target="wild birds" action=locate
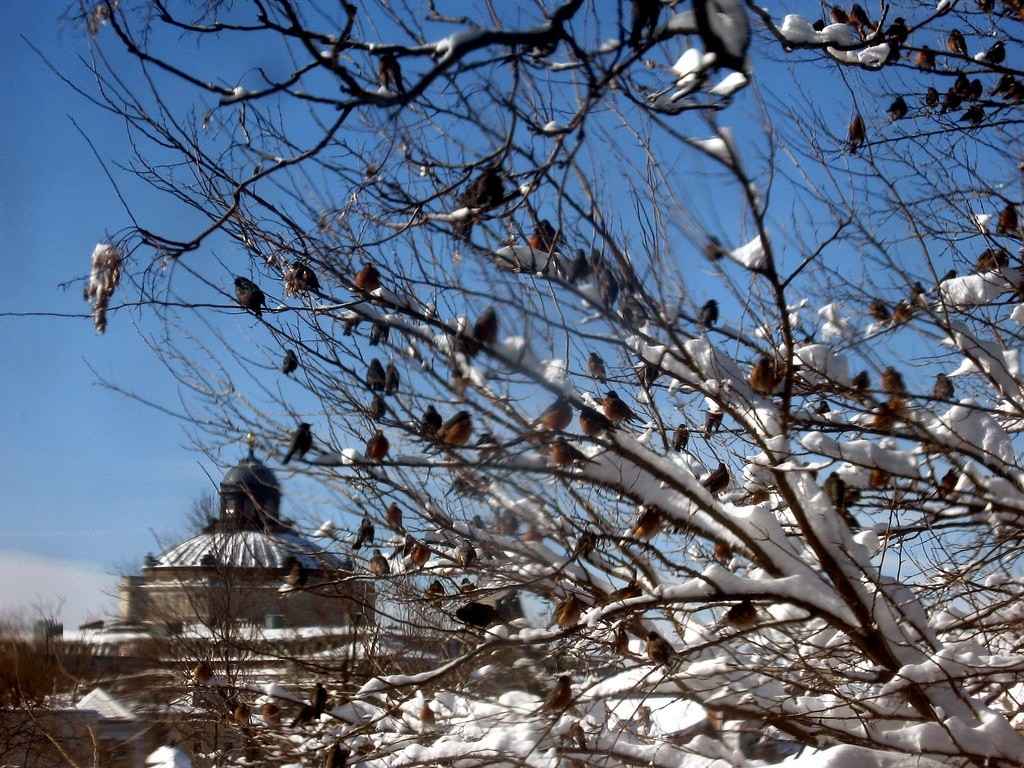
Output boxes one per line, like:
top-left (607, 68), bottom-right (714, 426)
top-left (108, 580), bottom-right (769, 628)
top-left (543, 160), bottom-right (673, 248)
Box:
top-left (231, 5), bottom-right (1023, 732)
top-left (193, 660), bottom-right (214, 686)
